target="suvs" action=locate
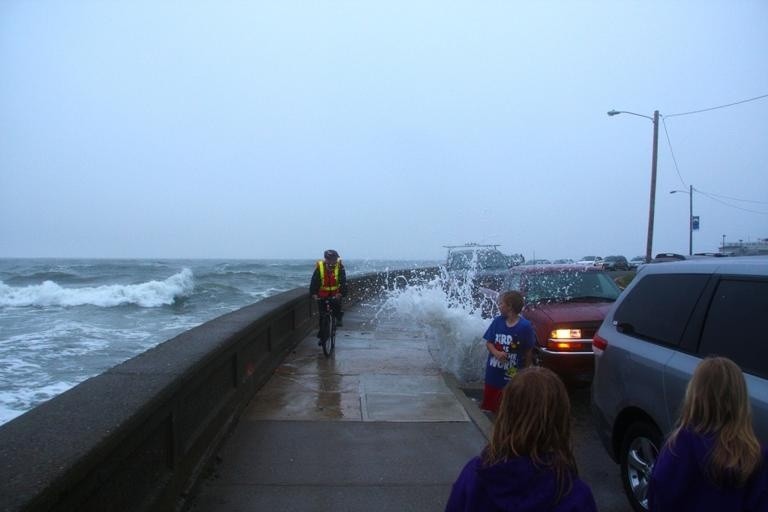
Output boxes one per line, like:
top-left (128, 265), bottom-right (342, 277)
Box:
top-left (592, 255), bottom-right (768, 512)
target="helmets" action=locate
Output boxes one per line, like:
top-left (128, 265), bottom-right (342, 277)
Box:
top-left (324, 250), bottom-right (339, 259)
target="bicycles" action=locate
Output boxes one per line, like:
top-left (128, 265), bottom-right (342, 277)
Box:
top-left (315, 297), bottom-right (336, 356)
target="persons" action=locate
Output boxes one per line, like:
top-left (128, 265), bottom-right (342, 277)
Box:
top-left (479, 290), bottom-right (536, 414)
top-left (310, 250), bottom-right (346, 344)
top-left (445, 366), bottom-right (597, 511)
top-left (648, 357), bottom-right (768, 511)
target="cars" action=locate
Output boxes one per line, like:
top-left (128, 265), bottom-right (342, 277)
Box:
top-left (446, 249), bottom-right (646, 375)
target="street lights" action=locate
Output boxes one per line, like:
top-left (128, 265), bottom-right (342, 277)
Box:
top-left (670, 190), bottom-right (692, 256)
top-left (608, 111), bottom-right (658, 263)
